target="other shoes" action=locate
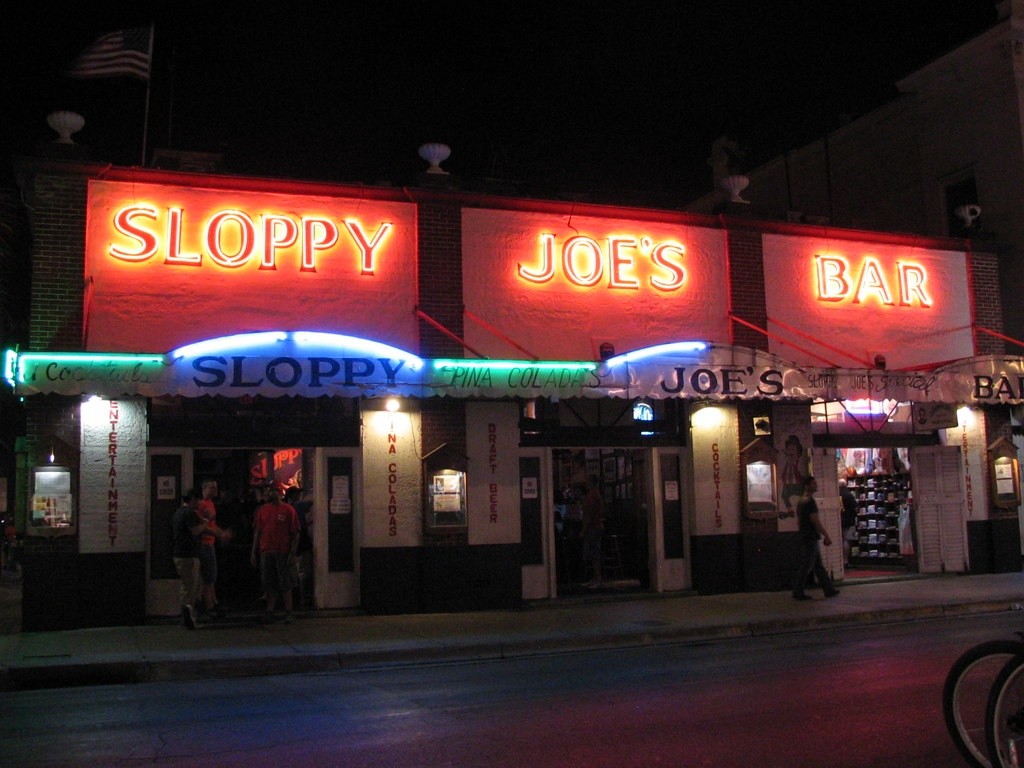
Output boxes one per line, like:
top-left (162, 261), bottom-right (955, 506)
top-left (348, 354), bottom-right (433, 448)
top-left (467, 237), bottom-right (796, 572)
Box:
top-left (265, 612), bottom-right (276, 623)
top-left (183, 603), bottom-right (199, 631)
top-left (284, 612), bottom-right (297, 623)
top-left (825, 589), bottom-right (841, 599)
top-left (208, 604), bottom-right (228, 622)
top-left (791, 592), bottom-right (812, 601)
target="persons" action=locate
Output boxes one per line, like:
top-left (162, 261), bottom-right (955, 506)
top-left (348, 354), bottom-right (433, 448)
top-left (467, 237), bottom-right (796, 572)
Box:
top-left (579, 475), bottom-right (604, 587)
top-left (791, 474), bottom-right (840, 600)
top-left (839, 479), bottom-right (856, 567)
top-left (172, 478), bottom-right (314, 629)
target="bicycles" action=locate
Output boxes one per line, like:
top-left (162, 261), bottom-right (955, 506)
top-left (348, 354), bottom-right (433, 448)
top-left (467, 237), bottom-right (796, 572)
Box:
top-left (941, 632), bottom-right (1024, 768)
top-left (982, 652), bottom-right (1024, 768)
